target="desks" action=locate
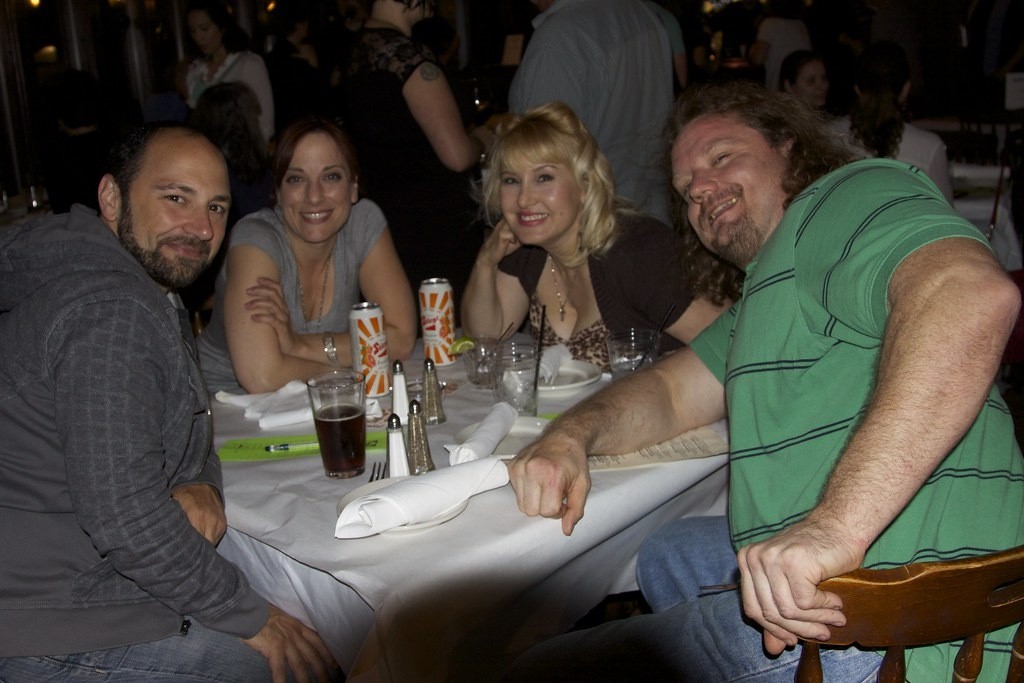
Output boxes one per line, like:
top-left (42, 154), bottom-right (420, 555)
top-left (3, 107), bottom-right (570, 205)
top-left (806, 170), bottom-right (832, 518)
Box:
top-left (910, 117), bottom-right (1021, 168)
top-left (939, 160), bottom-right (1023, 274)
top-left (185, 327), bottom-right (734, 683)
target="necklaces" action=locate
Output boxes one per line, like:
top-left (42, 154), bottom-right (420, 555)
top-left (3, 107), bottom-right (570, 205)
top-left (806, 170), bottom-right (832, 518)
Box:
top-left (551, 267), bottom-right (575, 322)
top-left (295, 255), bottom-right (332, 334)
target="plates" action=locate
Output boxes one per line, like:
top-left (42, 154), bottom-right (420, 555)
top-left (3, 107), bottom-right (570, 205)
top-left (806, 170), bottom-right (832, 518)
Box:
top-left (336, 474), bottom-right (470, 535)
top-left (536, 358), bottom-right (604, 398)
top-left (452, 416), bottom-right (552, 458)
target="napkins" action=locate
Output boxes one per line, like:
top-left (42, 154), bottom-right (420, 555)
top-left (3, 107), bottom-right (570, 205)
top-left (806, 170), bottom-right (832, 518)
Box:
top-left (332, 455), bottom-right (508, 541)
top-left (504, 344), bottom-right (573, 394)
top-left (242, 379), bottom-right (384, 429)
top-left (442, 400), bottom-right (517, 466)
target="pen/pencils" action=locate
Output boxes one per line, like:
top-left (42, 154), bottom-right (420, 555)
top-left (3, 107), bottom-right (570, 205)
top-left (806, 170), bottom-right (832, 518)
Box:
top-left (265, 442), bottom-right (319, 452)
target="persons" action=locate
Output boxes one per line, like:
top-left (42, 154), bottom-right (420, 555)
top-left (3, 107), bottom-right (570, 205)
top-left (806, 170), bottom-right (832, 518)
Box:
top-left (0, 122), bottom-right (347, 682)
top-left (824, 41), bottom-right (954, 206)
top-left (779, 51), bottom-right (828, 106)
top-left (199, 120), bottom-right (417, 395)
top-left (319, 0), bottom-right (500, 304)
top-left (750, 0), bottom-right (810, 91)
top-left (509, 1), bottom-right (706, 230)
top-left (184, 0), bottom-right (273, 149)
top-left (705, 0), bottom-right (765, 78)
top-left (266, 0), bottom-right (325, 136)
top-left (495, 76), bottom-right (1024, 683)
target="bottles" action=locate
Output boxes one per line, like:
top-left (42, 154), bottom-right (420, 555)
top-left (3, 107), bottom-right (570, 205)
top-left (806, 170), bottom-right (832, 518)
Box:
top-left (488, 345), bottom-right (539, 413)
top-left (386, 414), bottom-right (410, 479)
top-left (423, 357), bottom-right (446, 425)
top-left (392, 358), bottom-right (409, 425)
top-left (406, 400), bottom-right (436, 474)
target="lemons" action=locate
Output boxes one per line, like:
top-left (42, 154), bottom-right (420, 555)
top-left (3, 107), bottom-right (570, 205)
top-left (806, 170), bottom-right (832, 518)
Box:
top-left (448, 337), bottom-right (476, 355)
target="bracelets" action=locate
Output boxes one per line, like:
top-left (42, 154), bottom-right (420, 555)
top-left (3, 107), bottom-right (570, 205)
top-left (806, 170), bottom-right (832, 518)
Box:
top-left (323, 330), bottom-right (337, 362)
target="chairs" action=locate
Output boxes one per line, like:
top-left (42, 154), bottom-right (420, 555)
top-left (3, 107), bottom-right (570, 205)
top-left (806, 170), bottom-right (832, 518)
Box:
top-left (793, 545), bottom-right (1024, 683)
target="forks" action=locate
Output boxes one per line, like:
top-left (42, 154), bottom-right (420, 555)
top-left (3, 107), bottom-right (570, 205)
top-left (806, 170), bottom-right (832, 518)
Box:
top-left (368, 462), bottom-right (386, 482)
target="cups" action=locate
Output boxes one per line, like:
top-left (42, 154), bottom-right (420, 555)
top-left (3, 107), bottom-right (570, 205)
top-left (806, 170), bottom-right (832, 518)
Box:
top-left (605, 325), bottom-right (665, 382)
top-left (305, 370), bottom-right (367, 480)
top-left (465, 334), bottom-right (502, 387)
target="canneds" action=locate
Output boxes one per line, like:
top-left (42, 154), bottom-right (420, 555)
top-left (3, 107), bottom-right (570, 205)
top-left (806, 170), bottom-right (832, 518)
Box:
top-left (418, 278), bottom-right (457, 367)
top-left (348, 302), bottom-right (392, 397)
